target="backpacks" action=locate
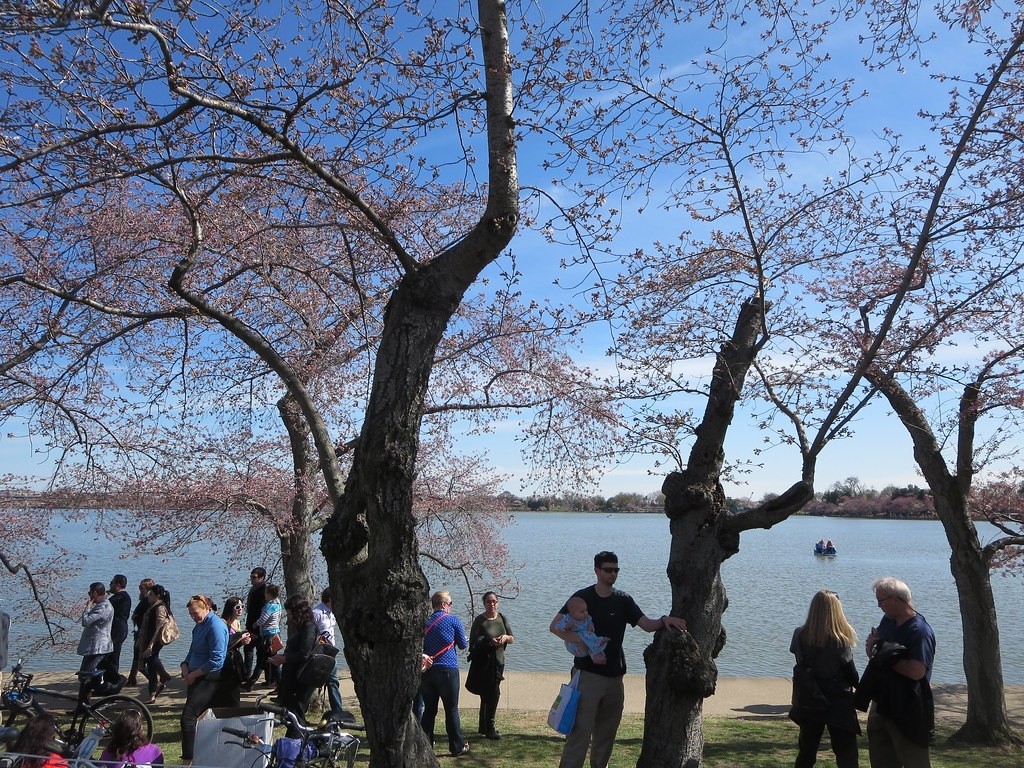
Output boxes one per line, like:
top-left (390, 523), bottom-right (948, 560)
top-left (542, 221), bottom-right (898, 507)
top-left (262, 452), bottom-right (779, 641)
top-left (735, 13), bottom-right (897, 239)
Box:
top-left (294, 621), bottom-right (339, 687)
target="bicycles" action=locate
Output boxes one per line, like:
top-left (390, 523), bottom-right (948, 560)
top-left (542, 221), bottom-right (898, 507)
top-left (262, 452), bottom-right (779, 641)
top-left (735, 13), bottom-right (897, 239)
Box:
top-left (814, 543), bottom-right (836, 555)
top-left (0, 658), bottom-right (153, 757)
top-left (221, 703), bottom-right (359, 768)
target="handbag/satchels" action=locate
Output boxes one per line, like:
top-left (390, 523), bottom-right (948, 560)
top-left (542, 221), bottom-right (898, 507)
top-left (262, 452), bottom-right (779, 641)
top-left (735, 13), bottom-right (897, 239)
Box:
top-left (547, 670), bottom-right (581, 736)
top-left (421, 654), bottom-right (433, 672)
top-left (155, 603), bottom-right (179, 646)
top-left (266, 634), bottom-right (284, 658)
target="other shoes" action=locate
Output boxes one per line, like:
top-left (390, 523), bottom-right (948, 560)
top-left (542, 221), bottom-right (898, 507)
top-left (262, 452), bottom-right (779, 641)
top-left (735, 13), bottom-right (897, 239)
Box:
top-left (144, 696), bottom-right (155, 704)
top-left (481, 730), bottom-right (500, 740)
top-left (125, 675), bottom-right (136, 686)
top-left (453, 742), bottom-right (470, 756)
top-left (155, 684), bottom-right (166, 699)
top-left (66, 708), bottom-right (92, 718)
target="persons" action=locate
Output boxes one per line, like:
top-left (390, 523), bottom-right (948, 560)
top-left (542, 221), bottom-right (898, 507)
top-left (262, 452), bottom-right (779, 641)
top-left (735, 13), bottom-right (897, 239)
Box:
top-left (11, 713), bottom-right (70, 768)
top-left (283, 586), bottom-right (343, 738)
top-left (413, 590), bottom-right (470, 757)
top-left (179, 593), bottom-right (229, 768)
top-left (0, 609), bottom-right (12, 670)
top-left (221, 567), bottom-right (283, 692)
top-left (548, 551), bottom-right (688, 768)
top-left (789, 590), bottom-right (860, 768)
top-left (813, 539), bottom-right (837, 556)
top-left (66, 574), bottom-right (170, 717)
top-left (99, 710), bottom-right (165, 768)
top-left (865, 578), bottom-right (938, 768)
top-left (468, 591), bottom-right (514, 741)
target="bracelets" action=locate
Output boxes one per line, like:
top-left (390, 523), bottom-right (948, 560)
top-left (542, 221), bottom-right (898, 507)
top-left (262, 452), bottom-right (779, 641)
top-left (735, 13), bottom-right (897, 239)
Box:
top-left (658, 613), bottom-right (668, 625)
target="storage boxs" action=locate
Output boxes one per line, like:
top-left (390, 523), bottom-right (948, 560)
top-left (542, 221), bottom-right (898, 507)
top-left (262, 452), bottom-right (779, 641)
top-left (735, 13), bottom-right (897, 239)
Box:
top-left (194, 707), bottom-right (275, 768)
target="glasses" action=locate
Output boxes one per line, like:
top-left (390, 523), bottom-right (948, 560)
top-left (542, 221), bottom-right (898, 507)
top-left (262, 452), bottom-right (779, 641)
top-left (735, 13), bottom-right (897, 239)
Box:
top-left (485, 601), bottom-right (498, 604)
top-left (250, 575), bottom-right (257, 580)
top-left (88, 592), bottom-right (93, 596)
top-left (191, 595), bottom-right (206, 606)
top-left (830, 591), bottom-right (839, 600)
top-left (232, 604), bottom-right (245, 610)
top-left (129, 709), bottom-right (141, 716)
top-left (444, 601), bottom-right (453, 605)
top-left (601, 567), bottom-right (620, 573)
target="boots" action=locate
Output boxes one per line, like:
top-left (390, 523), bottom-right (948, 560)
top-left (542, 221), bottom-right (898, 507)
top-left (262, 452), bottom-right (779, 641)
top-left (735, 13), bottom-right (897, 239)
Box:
top-left (158, 669), bottom-right (172, 684)
